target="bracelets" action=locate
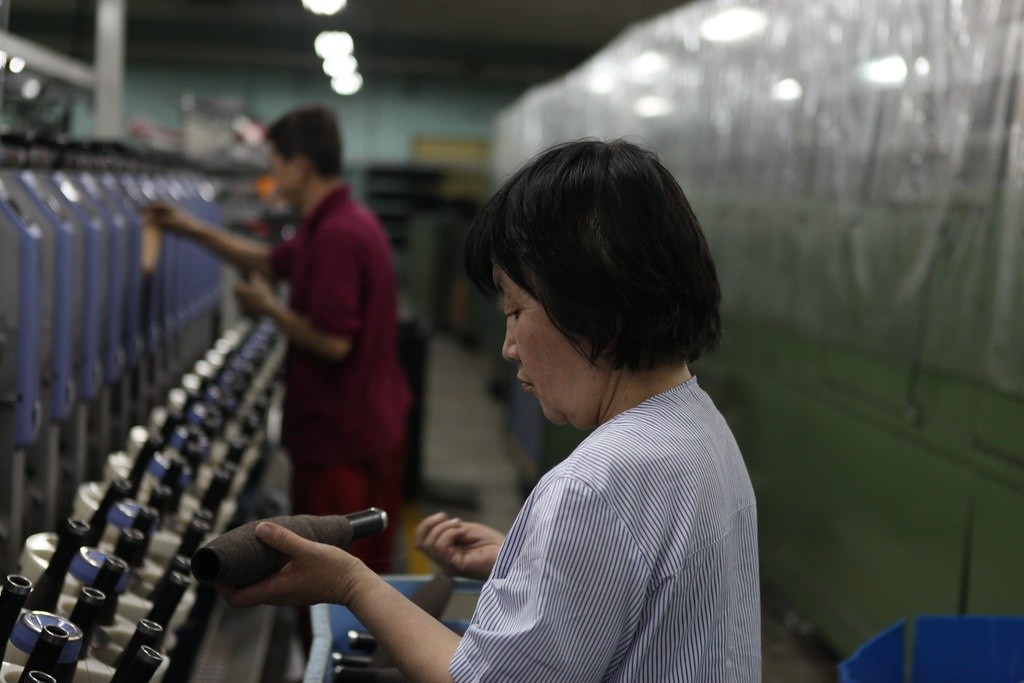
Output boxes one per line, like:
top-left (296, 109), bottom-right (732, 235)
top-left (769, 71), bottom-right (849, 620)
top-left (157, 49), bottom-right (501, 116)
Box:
top-left (269, 296), bottom-right (289, 327)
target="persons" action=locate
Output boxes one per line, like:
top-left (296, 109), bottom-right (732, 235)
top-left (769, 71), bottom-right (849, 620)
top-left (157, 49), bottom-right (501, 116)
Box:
top-left (146, 107), bottom-right (411, 683)
top-left (216, 138), bottom-right (762, 683)
